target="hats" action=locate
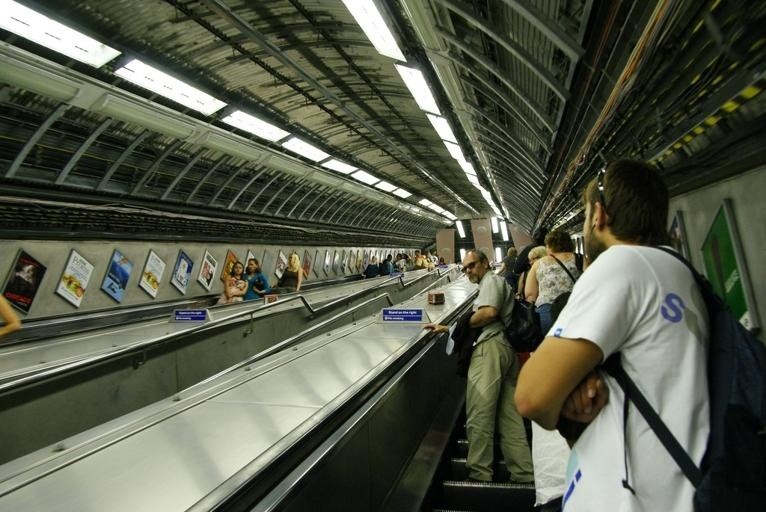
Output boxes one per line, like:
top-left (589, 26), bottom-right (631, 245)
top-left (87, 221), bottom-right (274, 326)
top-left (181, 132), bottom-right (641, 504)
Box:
top-left (533, 227), bottom-right (550, 243)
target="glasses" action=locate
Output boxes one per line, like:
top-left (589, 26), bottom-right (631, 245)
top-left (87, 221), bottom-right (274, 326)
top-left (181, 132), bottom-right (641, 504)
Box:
top-left (597, 159), bottom-right (611, 215)
top-left (461, 259), bottom-right (481, 273)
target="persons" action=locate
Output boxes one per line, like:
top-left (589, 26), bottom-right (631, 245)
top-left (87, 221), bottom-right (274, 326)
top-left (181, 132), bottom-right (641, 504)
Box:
top-left (273, 253), bottom-right (303, 293)
top-left (217, 261), bottom-right (248, 305)
top-left (0, 295), bottom-right (21, 337)
top-left (514, 161), bottom-right (710, 512)
top-left (424, 249), bottom-right (534, 483)
top-left (497, 227), bottom-right (589, 507)
top-left (243, 259), bottom-right (271, 300)
top-left (363, 249), bottom-right (448, 279)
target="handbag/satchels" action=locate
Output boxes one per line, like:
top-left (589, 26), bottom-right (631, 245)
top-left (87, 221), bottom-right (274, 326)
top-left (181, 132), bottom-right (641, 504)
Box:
top-left (694, 275), bottom-right (766, 511)
top-left (504, 297), bottom-right (543, 352)
top-left (254, 278), bottom-right (265, 297)
